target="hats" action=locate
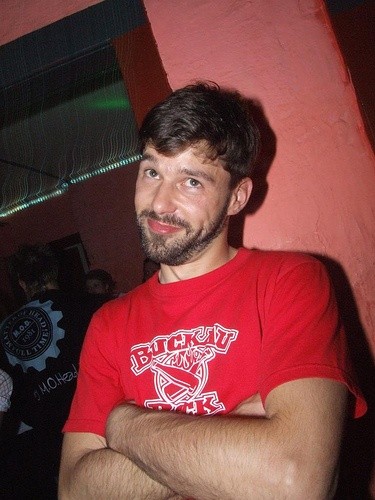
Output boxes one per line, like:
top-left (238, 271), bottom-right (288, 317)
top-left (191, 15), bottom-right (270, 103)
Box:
top-left (0, 368), bottom-right (13, 412)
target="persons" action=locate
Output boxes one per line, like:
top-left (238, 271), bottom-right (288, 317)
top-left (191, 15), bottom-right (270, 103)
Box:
top-left (1, 242), bottom-right (111, 445)
top-left (142, 257), bottom-right (161, 284)
top-left (59, 78), bottom-right (370, 500)
top-left (82, 267), bottom-right (129, 298)
